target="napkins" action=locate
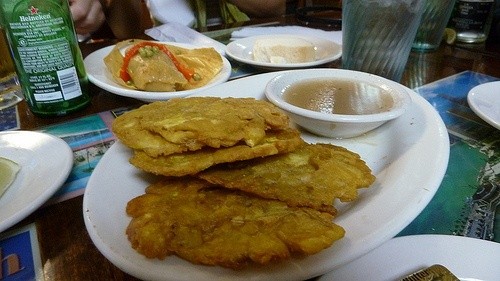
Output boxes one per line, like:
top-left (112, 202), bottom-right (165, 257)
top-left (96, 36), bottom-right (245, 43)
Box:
top-left (144, 20), bottom-right (227, 59)
top-left (230, 26), bottom-right (342, 46)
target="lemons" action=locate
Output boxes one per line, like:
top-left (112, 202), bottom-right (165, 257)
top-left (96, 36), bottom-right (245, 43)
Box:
top-left (0, 157), bottom-right (22, 197)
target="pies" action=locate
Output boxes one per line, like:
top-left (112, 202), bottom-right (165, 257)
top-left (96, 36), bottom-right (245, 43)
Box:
top-left (112, 96), bottom-right (374, 267)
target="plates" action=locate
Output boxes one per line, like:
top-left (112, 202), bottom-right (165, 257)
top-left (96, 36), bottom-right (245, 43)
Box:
top-left (467, 80), bottom-right (500, 130)
top-left (83, 41), bottom-right (232, 104)
top-left (81, 68), bottom-right (450, 281)
top-left (0, 130), bottom-right (74, 234)
top-left (224, 33), bottom-right (342, 71)
top-left (316, 233), bottom-right (500, 281)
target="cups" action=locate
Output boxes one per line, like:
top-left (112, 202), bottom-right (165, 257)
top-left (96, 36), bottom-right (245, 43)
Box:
top-left (340, 0), bottom-right (426, 84)
top-left (410, 0), bottom-right (456, 52)
top-left (450, 0), bottom-right (496, 44)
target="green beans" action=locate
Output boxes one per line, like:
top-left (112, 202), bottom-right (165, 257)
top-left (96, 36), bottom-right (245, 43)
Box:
top-left (127, 45), bottom-right (201, 90)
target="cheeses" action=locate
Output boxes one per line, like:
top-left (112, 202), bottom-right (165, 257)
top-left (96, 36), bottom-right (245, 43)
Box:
top-left (252, 35), bottom-right (314, 64)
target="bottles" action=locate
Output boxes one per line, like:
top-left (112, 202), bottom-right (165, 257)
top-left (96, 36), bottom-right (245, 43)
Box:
top-left (0, 0), bottom-right (94, 118)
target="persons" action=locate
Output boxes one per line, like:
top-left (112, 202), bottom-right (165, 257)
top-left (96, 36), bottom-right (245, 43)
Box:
top-left (0, 0), bottom-right (288, 73)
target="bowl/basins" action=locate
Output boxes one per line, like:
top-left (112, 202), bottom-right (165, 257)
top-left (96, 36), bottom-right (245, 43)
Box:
top-left (264, 68), bottom-right (413, 140)
